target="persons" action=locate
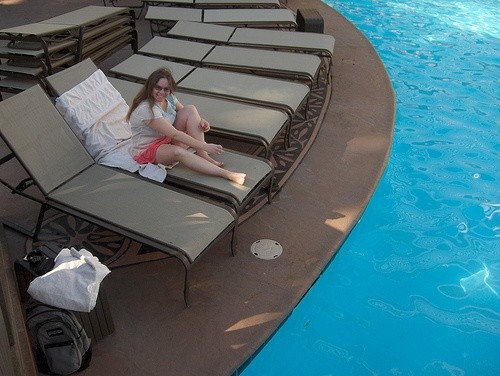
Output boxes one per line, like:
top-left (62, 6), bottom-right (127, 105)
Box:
top-left (123, 67), bottom-right (247, 185)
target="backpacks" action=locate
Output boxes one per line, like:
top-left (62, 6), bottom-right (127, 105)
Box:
top-left (23, 298), bottom-right (92, 376)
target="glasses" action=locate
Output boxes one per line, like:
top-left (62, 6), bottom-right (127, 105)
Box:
top-left (153, 85), bottom-right (171, 92)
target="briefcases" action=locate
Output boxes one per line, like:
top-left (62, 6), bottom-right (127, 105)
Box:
top-left (14, 242), bottom-right (114, 344)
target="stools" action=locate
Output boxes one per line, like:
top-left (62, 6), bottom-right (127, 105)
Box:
top-left (15, 251), bottom-right (115, 347)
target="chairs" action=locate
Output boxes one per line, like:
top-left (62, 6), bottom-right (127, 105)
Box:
top-left (0, 0), bottom-right (336, 167)
top-left (0, 83), bottom-right (236, 308)
top-left (46, 58), bottom-right (274, 255)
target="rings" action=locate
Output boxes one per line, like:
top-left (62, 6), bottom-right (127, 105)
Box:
top-left (214, 153), bottom-right (215, 155)
